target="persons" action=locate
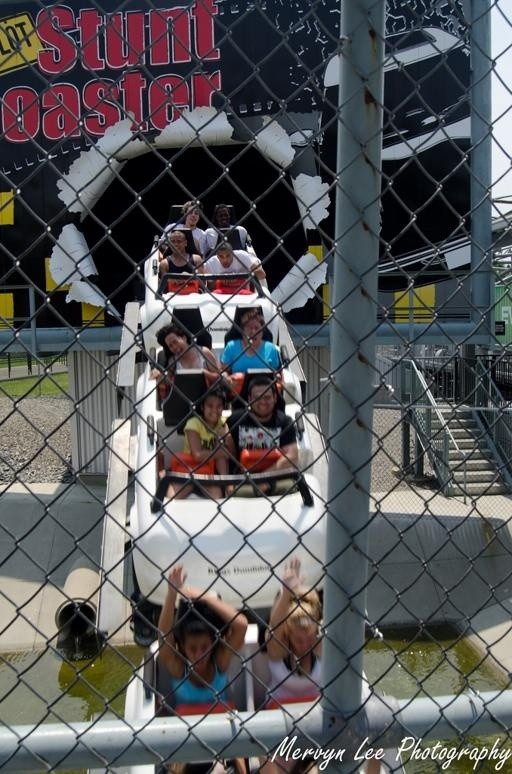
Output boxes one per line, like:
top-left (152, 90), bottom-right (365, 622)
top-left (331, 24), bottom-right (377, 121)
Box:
top-left (222, 310), bottom-right (281, 376)
top-left (200, 202), bottom-right (251, 248)
top-left (151, 322), bottom-right (216, 400)
top-left (166, 199), bottom-right (208, 251)
top-left (159, 226), bottom-right (205, 277)
top-left (259, 554), bottom-right (381, 773)
top-left (154, 562), bottom-right (251, 773)
top-left (171, 387), bottom-right (236, 498)
top-left (227, 377), bottom-right (302, 498)
top-left (204, 240), bottom-right (266, 277)
